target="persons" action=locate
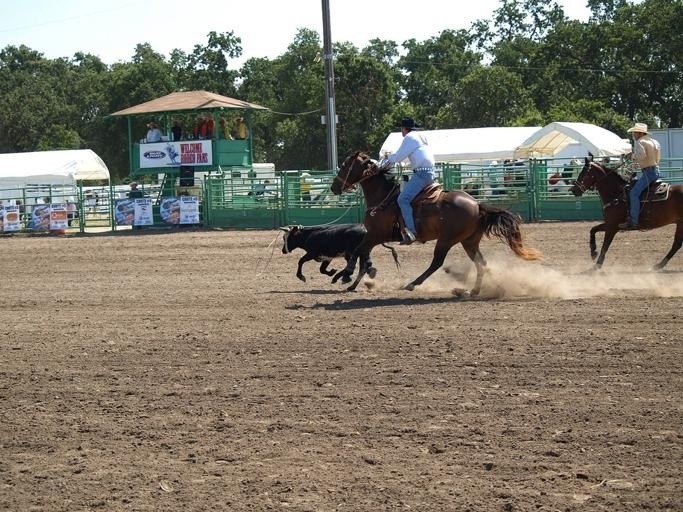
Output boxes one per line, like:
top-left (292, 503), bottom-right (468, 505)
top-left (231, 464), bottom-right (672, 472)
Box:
top-left (143, 115), bottom-right (249, 144)
top-left (248, 179), bottom-right (279, 209)
top-left (123, 211), bottom-right (135, 224)
top-left (115, 204), bottom-right (127, 225)
top-left (0, 187), bottom-right (99, 232)
top-left (299, 172), bottom-right (319, 208)
top-left (169, 145), bottom-right (176, 158)
top-left (163, 143), bottom-right (176, 166)
top-left (460, 156), bottom-right (642, 200)
top-left (127, 181), bottom-right (144, 230)
top-left (162, 200), bottom-right (174, 222)
top-left (365, 117), bottom-right (437, 246)
top-left (169, 211), bottom-right (179, 225)
top-left (618, 122), bottom-right (661, 230)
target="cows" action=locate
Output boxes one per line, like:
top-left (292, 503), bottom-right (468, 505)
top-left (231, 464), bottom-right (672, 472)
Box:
top-left (278, 223), bottom-right (401, 284)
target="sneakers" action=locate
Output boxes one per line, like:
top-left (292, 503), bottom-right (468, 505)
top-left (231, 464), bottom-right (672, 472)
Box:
top-left (618, 222), bottom-right (639, 230)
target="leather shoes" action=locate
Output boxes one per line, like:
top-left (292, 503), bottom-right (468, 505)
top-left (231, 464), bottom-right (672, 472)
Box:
top-left (399, 234), bottom-right (415, 245)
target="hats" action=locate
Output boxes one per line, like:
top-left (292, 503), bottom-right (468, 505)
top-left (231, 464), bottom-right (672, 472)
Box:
top-left (147, 121), bottom-right (157, 127)
top-left (395, 119), bottom-right (422, 129)
top-left (129, 182), bottom-right (138, 186)
top-left (233, 116), bottom-right (243, 122)
top-left (627, 123), bottom-right (651, 134)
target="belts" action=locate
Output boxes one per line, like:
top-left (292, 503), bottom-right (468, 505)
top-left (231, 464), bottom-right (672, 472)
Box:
top-left (641, 165), bottom-right (658, 172)
top-left (414, 168), bottom-right (434, 172)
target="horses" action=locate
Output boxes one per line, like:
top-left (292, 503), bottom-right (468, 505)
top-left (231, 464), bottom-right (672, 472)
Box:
top-left (571, 152), bottom-right (683, 270)
top-left (330, 150), bottom-right (547, 298)
